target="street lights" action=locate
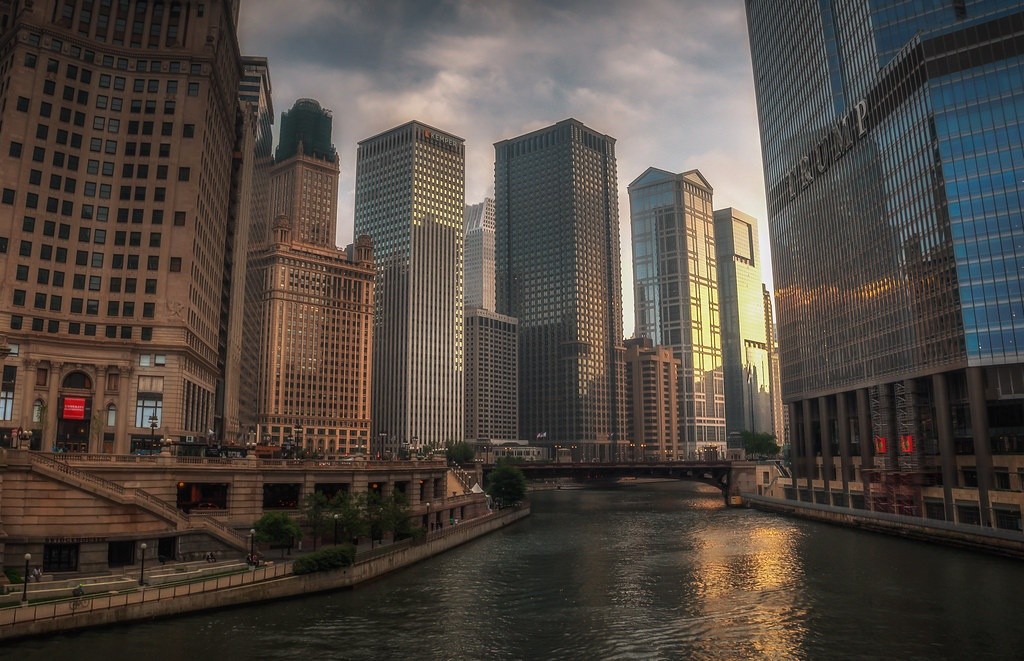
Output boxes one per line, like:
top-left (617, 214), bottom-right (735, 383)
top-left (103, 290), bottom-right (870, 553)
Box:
top-left (379, 430), bottom-right (387, 461)
top-left (22, 553), bottom-right (31, 601)
top-left (334, 514), bottom-right (338, 546)
top-left (148, 409), bottom-right (160, 458)
top-left (250, 529), bottom-right (256, 566)
top-left (293, 423), bottom-right (302, 460)
top-left (139, 543), bottom-right (146, 586)
top-left (427, 502), bottom-right (430, 533)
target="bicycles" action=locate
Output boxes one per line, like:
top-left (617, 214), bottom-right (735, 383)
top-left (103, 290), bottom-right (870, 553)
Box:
top-left (69, 592), bottom-right (90, 608)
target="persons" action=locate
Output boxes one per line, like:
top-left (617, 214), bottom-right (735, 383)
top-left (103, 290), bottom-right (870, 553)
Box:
top-left (246, 553), bottom-right (259, 567)
top-left (430, 521), bottom-right (434, 531)
top-left (436, 521), bottom-right (438, 530)
top-left (33, 566), bottom-right (44, 581)
top-left (205, 552), bottom-right (217, 563)
top-left (450, 517), bottom-right (458, 525)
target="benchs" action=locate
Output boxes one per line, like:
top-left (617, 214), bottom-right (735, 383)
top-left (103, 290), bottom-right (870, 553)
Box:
top-left (0, 559), bottom-right (264, 602)
